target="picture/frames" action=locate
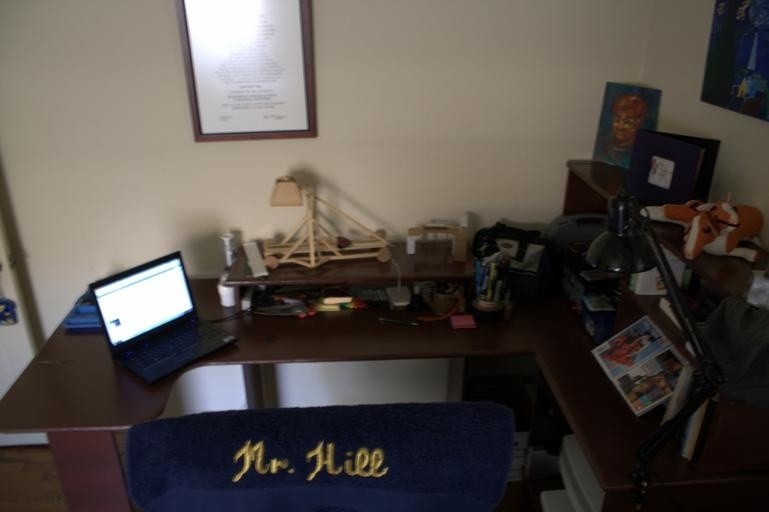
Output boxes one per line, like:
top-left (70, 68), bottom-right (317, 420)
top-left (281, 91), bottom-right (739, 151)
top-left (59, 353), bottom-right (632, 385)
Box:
top-left (173, 0), bottom-right (319, 143)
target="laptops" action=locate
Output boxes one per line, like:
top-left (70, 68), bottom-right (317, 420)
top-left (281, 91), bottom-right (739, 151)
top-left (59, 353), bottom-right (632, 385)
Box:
top-left (89, 251), bottom-right (242, 385)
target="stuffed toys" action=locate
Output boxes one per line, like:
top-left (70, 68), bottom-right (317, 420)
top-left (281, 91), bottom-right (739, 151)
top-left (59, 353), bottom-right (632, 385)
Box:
top-left (641, 199), bottom-right (765, 263)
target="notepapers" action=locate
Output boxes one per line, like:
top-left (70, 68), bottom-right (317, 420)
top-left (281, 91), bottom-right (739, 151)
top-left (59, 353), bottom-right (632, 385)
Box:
top-left (450, 314), bottom-right (476, 329)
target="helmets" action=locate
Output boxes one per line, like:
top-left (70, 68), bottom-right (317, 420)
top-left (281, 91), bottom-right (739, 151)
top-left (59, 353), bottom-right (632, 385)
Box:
top-left (612, 95), bottom-right (648, 118)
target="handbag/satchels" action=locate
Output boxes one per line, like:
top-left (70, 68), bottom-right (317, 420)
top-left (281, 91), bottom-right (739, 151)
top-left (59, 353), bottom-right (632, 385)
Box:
top-left (474, 227), bottom-right (564, 302)
top-left (700, 297), bottom-right (768, 405)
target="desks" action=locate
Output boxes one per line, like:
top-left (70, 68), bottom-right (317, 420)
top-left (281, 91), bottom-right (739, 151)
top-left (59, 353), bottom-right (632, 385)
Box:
top-left (0, 276), bottom-right (769, 512)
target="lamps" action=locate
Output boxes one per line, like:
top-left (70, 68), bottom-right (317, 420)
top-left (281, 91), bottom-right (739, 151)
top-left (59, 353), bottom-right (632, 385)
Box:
top-left (587, 195), bottom-right (726, 512)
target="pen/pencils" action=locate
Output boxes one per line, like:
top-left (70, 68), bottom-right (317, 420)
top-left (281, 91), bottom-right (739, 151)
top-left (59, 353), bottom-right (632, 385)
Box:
top-left (476, 260), bottom-right (482, 298)
top-left (481, 267), bottom-right (490, 298)
top-left (487, 263), bottom-right (498, 300)
top-left (379, 317), bottom-right (421, 326)
top-left (493, 280), bottom-right (503, 301)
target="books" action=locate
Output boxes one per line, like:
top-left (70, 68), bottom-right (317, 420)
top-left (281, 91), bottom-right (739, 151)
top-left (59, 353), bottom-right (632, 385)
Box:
top-left (591, 314), bottom-right (692, 418)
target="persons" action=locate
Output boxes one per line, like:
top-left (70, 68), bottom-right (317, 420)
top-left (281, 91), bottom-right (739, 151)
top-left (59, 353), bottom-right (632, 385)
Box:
top-left (625, 334), bottom-right (655, 357)
top-left (593, 94), bottom-right (650, 169)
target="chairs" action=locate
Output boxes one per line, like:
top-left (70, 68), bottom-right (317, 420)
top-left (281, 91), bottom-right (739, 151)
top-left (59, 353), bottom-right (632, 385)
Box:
top-left (127, 401), bottom-right (518, 512)
